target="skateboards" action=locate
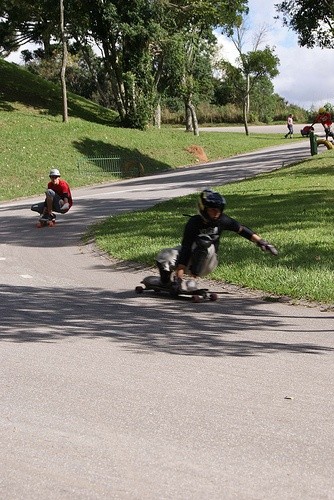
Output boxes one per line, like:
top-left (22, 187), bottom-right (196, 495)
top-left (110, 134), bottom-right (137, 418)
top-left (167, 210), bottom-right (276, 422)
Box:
top-left (136, 276), bottom-right (229, 303)
top-left (36, 214), bottom-right (56, 228)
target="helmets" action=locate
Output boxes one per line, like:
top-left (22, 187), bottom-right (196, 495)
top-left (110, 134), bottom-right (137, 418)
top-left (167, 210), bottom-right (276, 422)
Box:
top-left (199, 191), bottom-right (226, 220)
top-left (319, 108), bottom-right (326, 115)
top-left (50, 169), bottom-right (61, 175)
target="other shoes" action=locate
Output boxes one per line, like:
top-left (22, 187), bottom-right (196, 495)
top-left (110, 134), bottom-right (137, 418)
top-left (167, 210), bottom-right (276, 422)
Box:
top-left (326, 131), bottom-right (328, 139)
top-left (188, 241), bottom-right (208, 277)
top-left (331, 132), bottom-right (334, 139)
top-left (153, 261), bottom-right (172, 287)
top-left (42, 214), bottom-right (51, 218)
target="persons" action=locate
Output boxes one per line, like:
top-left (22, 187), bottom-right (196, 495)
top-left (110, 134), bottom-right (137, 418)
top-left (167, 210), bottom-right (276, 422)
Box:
top-left (157, 189), bottom-right (280, 293)
top-left (315, 107), bottom-right (334, 141)
top-left (31, 169), bottom-right (73, 222)
top-left (285, 114), bottom-right (294, 139)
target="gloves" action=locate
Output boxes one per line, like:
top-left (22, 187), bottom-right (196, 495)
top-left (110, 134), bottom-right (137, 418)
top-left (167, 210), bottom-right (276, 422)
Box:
top-left (171, 277), bottom-right (182, 290)
top-left (61, 203), bottom-right (69, 209)
top-left (256, 239), bottom-right (278, 256)
